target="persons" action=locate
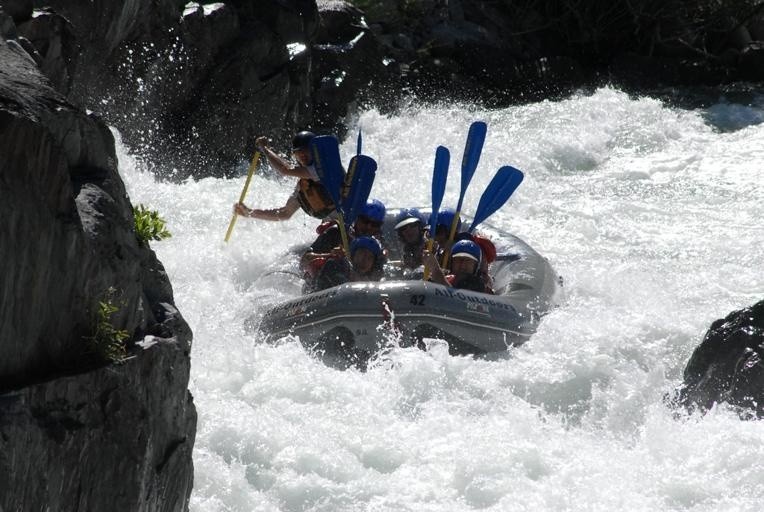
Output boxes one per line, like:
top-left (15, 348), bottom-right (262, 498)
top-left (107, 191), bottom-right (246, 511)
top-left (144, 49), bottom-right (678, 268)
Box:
top-left (308, 234), bottom-right (387, 292)
top-left (229, 129), bottom-right (356, 265)
top-left (418, 238), bottom-right (496, 293)
top-left (296, 197), bottom-right (386, 278)
top-left (392, 206), bottom-right (447, 282)
top-left (420, 208), bottom-right (489, 273)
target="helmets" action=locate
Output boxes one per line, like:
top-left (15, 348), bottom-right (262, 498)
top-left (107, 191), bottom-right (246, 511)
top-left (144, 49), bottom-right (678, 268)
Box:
top-left (427, 211), bottom-right (463, 231)
top-left (392, 206), bottom-right (425, 230)
top-left (291, 131), bottom-right (319, 153)
top-left (450, 233), bottom-right (497, 264)
top-left (356, 197), bottom-right (386, 222)
top-left (350, 236), bottom-right (380, 260)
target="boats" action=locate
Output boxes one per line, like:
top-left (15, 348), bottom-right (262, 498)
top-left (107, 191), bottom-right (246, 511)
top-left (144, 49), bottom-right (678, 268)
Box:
top-left (234, 211), bottom-right (555, 370)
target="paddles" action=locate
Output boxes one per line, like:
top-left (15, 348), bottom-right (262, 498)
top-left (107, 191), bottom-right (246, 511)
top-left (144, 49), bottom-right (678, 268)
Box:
top-left (444, 121), bottom-right (487, 270)
top-left (469, 165), bottom-right (524, 235)
top-left (310, 135), bottom-right (352, 260)
top-left (338, 154), bottom-right (377, 256)
top-left (423, 146), bottom-right (450, 282)
